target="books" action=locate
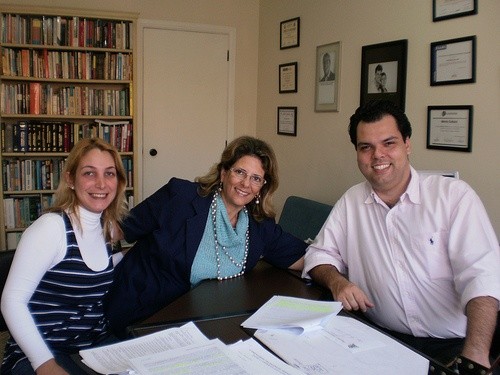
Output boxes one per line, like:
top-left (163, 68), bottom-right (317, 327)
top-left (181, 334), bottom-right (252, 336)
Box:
top-left (0, 11), bottom-right (136, 252)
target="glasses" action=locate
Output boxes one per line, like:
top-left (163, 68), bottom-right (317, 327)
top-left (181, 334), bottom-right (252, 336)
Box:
top-left (228, 167), bottom-right (265, 187)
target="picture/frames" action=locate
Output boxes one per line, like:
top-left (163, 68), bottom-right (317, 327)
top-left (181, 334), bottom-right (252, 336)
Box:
top-left (279, 61), bottom-right (297, 93)
top-left (279, 17), bottom-right (300, 50)
top-left (426, 104), bottom-right (474, 153)
top-left (313, 40), bottom-right (343, 112)
top-left (432, 0), bottom-right (478, 22)
top-left (277, 106), bottom-right (297, 136)
top-left (359, 38), bottom-right (408, 110)
top-left (430, 35), bottom-right (478, 86)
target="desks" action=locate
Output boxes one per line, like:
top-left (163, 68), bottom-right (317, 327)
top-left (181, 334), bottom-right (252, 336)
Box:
top-left (126, 262), bottom-right (453, 375)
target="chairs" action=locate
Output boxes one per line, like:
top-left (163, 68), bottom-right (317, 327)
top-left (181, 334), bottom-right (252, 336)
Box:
top-left (278, 195), bottom-right (338, 246)
top-left (0, 249), bottom-right (18, 331)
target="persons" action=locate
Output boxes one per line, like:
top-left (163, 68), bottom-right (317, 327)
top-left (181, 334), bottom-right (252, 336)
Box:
top-left (103, 134), bottom-right (309, 335)
top-left (0, 138), bottom-right (135, 375)
top-left (319, 54), bottom-right (336, 81)
top-left (367, 65), bottom-right (389, 93)
top-left (300, 101), bottom-right (500, 375)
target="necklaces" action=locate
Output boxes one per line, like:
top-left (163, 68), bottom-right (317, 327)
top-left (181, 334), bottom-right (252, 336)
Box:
top-left (210, 186), bottom-right (252, 281)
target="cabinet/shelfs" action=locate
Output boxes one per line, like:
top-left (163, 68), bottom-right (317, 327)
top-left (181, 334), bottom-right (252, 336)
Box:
top-left (0, 4), bottom-right (140, 252)
